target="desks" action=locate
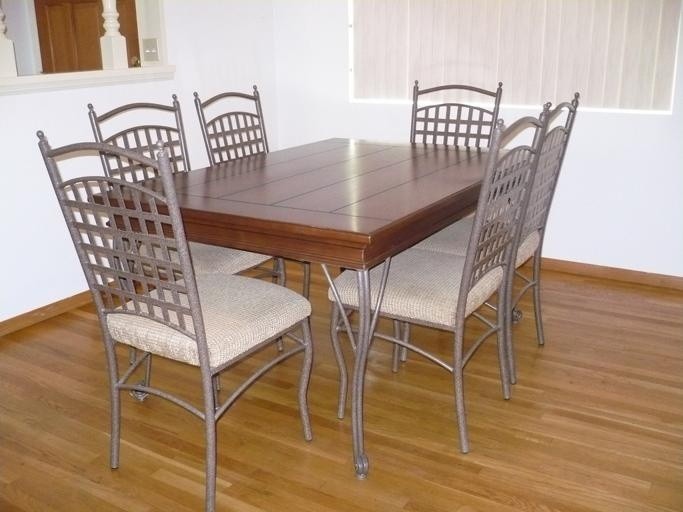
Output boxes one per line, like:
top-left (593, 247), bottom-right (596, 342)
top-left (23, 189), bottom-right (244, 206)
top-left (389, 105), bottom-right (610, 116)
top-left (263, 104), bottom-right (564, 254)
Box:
top-left (90, 137), bottom-right (523, 482)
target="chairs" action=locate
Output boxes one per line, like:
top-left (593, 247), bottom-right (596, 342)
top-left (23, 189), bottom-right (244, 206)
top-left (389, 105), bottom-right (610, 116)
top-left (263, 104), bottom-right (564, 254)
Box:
top-left (410, 82), bottom-right (503, 151)
top-left (36, 130), bottom-right (312, 509)
top-left (193, 84), bottom-right (311, 300)
top-left (397, 93), bottom-right (577, 385)
top-left (327, 103), bottom-right (551, 456)
top-left (88, 95), bottom-right (287, 365)
